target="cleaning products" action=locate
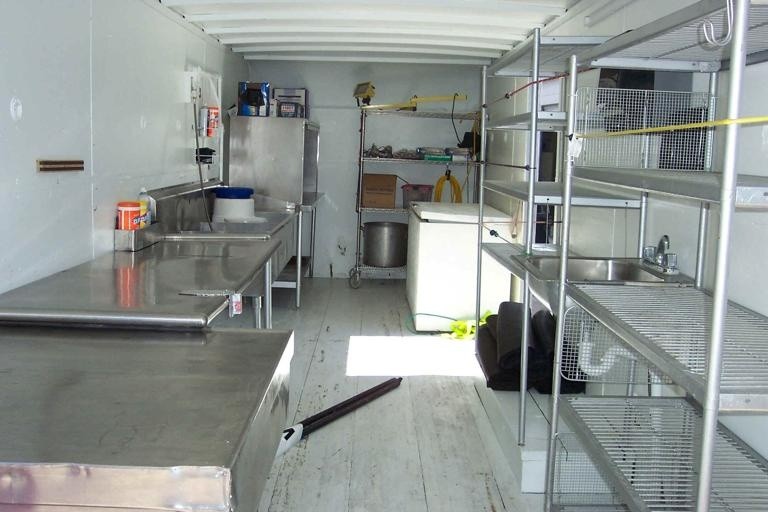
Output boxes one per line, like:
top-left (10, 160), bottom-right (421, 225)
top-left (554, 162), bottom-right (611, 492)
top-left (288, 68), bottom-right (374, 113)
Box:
top-left (138, 187), bottom-right (151, 229)
top-left (118, 201), bottom-right (140, 231)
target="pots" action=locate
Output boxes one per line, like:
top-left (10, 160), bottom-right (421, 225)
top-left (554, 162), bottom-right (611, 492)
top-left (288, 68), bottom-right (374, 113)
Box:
top-left (359, 221), bottom-right (410, 269)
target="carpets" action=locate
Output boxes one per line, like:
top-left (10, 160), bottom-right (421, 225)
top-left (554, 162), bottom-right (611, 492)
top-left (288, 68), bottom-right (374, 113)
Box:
top-left (345, 333), bottom-right (490, 377)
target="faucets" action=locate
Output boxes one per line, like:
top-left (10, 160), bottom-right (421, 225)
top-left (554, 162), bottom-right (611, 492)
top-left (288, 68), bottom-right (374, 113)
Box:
top-left (653, 234), bottom-right (670, 266)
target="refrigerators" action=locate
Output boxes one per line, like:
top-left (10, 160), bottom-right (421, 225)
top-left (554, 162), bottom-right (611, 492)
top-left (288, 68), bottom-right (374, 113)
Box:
top-left (403, 199), bottom-right (514, 334)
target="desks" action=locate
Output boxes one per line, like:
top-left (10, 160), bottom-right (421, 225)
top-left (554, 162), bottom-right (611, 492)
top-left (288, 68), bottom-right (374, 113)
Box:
top-left (0, 208), bottom-right (299, 330)
top-left (0, 328), bottom-right (297, 510)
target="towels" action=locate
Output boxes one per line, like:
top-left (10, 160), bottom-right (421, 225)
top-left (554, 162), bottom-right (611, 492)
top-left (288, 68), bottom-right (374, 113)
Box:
top-left (476, 301), bottom-right (586, 396)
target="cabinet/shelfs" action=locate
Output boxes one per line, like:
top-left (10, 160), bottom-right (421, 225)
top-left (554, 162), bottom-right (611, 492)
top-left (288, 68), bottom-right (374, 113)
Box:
top-left (348, 98), bottom-right (482, 288)
top-left (484, 0), bottom-right (768, 512)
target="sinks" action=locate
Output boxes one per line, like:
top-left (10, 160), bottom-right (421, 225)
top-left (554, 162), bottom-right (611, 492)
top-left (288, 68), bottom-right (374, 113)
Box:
top-left (510, 252), bottom-right (697, 286)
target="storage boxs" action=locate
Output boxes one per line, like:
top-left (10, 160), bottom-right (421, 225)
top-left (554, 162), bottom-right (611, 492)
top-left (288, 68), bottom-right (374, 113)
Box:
top-left (360, 172), bottom-right (399, 210)
